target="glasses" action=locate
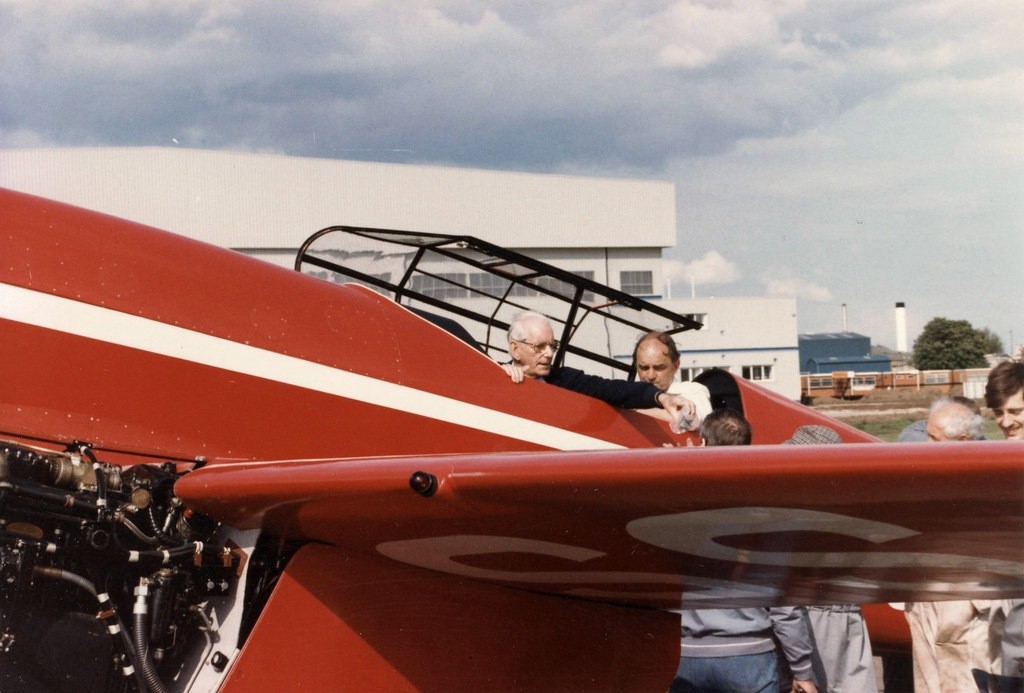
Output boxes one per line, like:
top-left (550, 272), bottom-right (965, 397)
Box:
top-left (515, 340), bottom-right (560, 353)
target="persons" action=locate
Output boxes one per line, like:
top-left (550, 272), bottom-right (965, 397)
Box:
top-left (501, 311), bottom-right (1023, 693)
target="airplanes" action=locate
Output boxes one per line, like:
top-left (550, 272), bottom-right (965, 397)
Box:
top-left (0, 183), bottom-right (1024, 693)
